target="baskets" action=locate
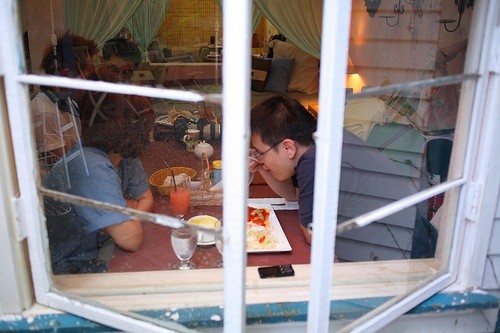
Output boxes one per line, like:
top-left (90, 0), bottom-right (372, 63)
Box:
top-left (149, 167), bottom-right (196, 197)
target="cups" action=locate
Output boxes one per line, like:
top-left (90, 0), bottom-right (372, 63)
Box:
top-left (182, 129), bottom-right (201, 153)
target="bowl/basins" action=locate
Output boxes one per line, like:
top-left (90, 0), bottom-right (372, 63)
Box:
top-left (148, 167), bottom-right (197, 196)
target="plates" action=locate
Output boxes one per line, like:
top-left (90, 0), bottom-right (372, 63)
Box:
top-left (187, 215), bottom-right (221, 246)
top-left (246, 204), bottom-right (293, 253)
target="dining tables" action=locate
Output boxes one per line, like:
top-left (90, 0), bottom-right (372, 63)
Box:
top-left (109, 140), bottom-right (339, 272)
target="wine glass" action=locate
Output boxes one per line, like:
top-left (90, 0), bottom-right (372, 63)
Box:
top-left (170, 176), bottom-right (190, 221)
top-left (216, 219), bottom-right (224, 269)
top-left (169, 225), bottom-right (199, 270)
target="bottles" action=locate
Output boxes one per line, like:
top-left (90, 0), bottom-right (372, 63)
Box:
top-left (211, 160), bottom-right (222, 185)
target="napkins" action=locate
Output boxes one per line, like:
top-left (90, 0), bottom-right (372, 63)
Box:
top-left (247, 198), bottom-right (300, 210)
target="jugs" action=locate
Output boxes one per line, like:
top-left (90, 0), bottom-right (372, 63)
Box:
top-left (194, 139), bottom-right (214, 161)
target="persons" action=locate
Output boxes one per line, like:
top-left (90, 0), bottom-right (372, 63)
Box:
top-left (250, 96), bottom-right (436, 262)
top-left (30, 31), bottom-right (155, 274)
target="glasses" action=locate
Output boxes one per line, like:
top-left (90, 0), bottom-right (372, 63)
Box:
top-left (254, 140), bottom-right (282, 161)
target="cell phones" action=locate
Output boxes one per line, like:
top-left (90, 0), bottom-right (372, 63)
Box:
top-left (257, 264), bottom-right (294, 279)
